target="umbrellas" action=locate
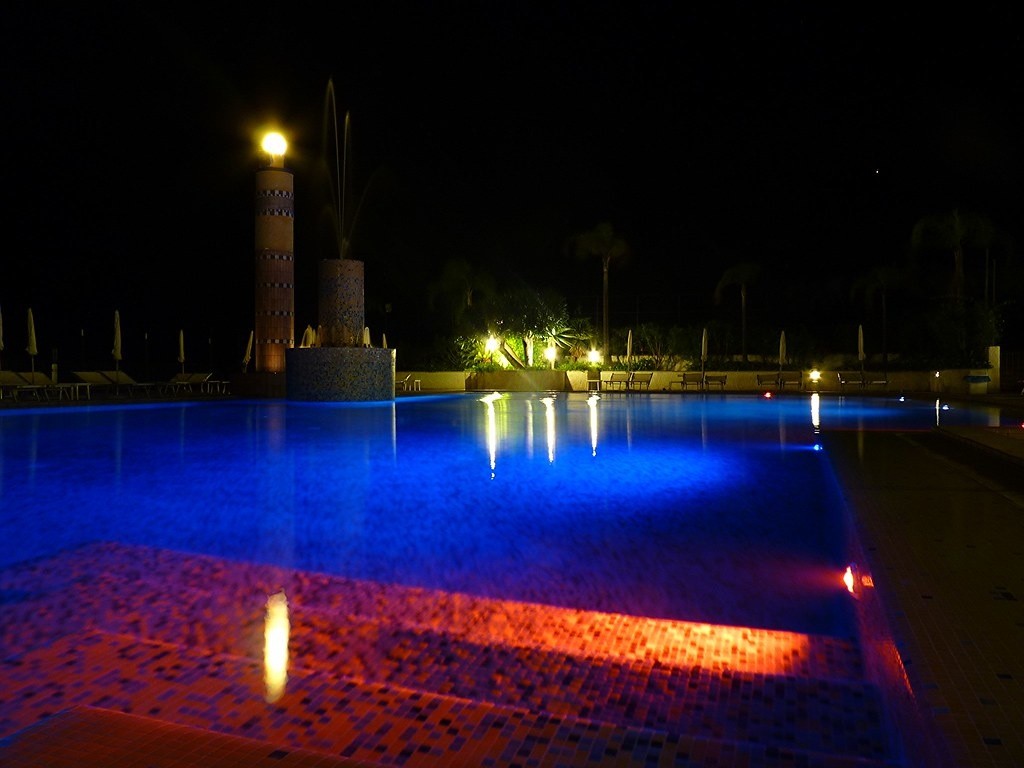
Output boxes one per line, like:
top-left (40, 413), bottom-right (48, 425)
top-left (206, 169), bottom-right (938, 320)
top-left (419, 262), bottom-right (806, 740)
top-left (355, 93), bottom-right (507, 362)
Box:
top-left (779, 331), bottom-right (786, 391)
top-left (700, 328), bottom-right (708, 391)
top-left (858, 325), bottom-right (866, 372)
top-left (112, 311), bottom-right (122, 395)
top-left (24, 308), bottom-right (38, 386)
top-left (241, 331), bottom-right (253, 377)
top-left (626, 330), bottom-right (632, 379)
top-left (178, 329), bottom-right (185, 380)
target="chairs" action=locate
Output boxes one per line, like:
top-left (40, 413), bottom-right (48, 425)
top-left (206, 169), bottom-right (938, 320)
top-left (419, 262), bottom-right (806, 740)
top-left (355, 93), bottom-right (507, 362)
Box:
top-left (839, 370), bottom-right (892, 393)
top-left (0, 371), bottom-right (232, 403)
top-left (394, 372), bottom-right (412, 392)
top-left (605, 373), bottom-right (654, 392)
top-left (757, 371), bottom-right (803, 395)
top-left (683, 372), bottom-right (729, 393)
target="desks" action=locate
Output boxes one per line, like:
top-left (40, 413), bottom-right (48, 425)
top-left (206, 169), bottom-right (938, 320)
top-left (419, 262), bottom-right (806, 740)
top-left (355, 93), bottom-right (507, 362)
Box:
top-left (670, 380), bottom-right (685, 392)
top-left (586, 379), bottom-right (602, 391)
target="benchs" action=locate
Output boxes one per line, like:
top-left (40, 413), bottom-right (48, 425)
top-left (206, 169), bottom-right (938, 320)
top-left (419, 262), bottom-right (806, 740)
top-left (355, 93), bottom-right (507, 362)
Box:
top-left (409, 380), bottom-right (421, 392)
